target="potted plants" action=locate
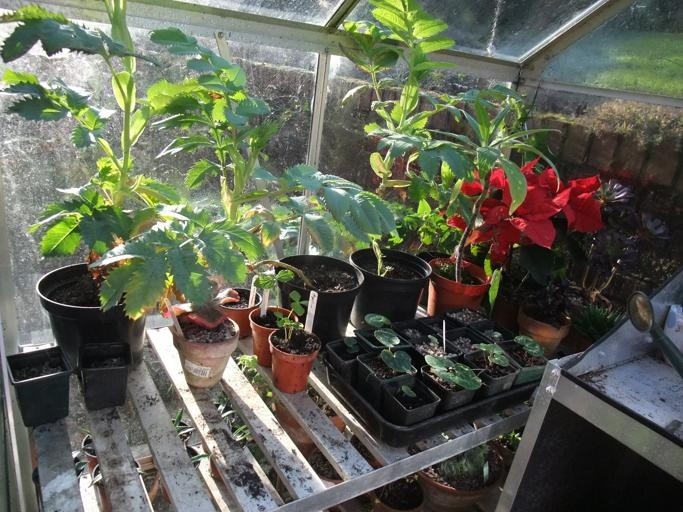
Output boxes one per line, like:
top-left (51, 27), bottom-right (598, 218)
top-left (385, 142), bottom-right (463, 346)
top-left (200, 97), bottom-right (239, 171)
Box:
top-left (414, 425), bottom-right (504, 510)
top-left (0, 1), bottom-right (633, 426)
